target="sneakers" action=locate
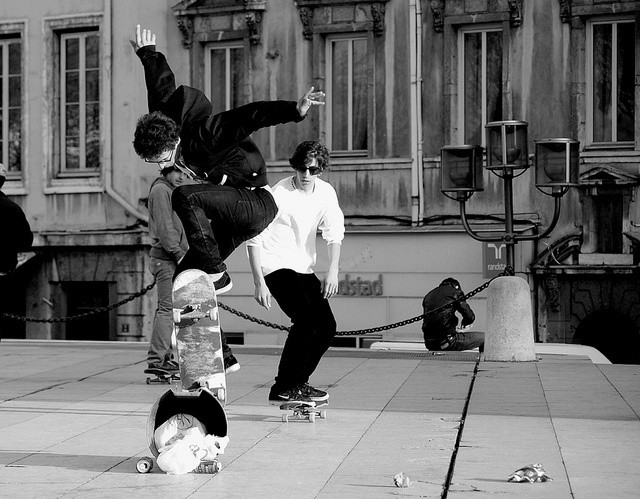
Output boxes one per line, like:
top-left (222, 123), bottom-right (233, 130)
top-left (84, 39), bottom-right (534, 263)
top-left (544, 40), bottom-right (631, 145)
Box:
top-left (222, 354), bottom-right (242, 375)
top-left (149, 358), bottom-right (179, 370)
top-left (301, 383), bottom-right (329, 401)
top-left (209, 271), bottom-right (232, 295)
top-left (268, 389), bottom-right (317, 406)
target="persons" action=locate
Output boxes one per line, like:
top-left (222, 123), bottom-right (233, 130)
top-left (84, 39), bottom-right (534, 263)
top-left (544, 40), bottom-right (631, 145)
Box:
top-left (244, 141), bottom-right (347, 406)
top-left (127, 25), bottom-right (328, 377)
top-left (147, 166), bottom-right (192, 376)
top-left (0, 163), bottom-right (34, 301)
top-left (423, 277), bottom-right (486, 352)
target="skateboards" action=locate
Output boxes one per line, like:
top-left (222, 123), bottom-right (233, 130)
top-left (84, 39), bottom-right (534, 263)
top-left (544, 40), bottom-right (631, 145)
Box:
top-left (171, 268), bottom-right (228, 409)
top-left (144, 368), bottom-right (181, 385)
top-left (279, 399), bottom-right (329, 423)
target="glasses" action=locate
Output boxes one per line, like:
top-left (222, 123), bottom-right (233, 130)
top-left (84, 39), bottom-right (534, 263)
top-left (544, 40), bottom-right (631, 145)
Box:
top-left (293, 164), bottom-right (322, 175)
top-left (145, 149), bottom-right (173, 163)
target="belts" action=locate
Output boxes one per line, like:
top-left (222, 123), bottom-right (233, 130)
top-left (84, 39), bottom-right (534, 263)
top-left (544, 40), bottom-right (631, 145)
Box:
top-left (440, 335), bottom-right (455, 349)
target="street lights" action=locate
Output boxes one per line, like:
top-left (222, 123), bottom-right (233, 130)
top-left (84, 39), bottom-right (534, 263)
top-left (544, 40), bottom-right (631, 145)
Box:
top-left (439, 120), bottom-right (580, 363)
